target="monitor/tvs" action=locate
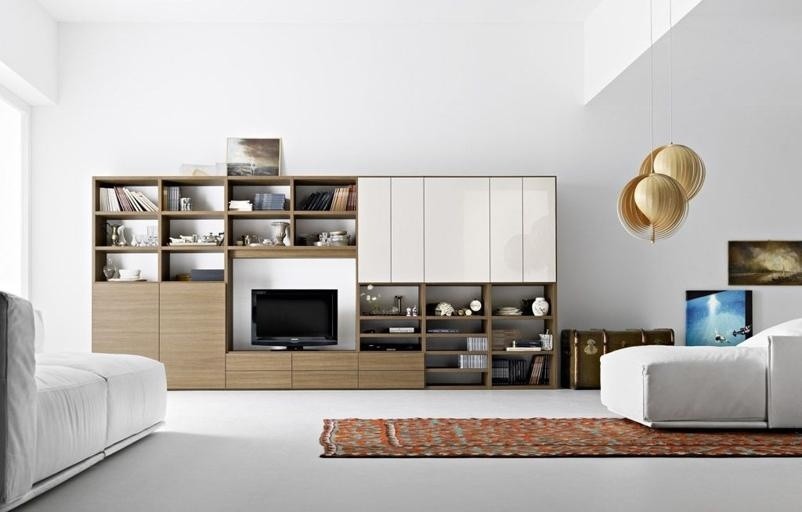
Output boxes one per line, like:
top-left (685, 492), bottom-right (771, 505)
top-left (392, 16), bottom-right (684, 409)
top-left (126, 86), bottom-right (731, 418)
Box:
top-left (100, 185), bottom-right (357, 211)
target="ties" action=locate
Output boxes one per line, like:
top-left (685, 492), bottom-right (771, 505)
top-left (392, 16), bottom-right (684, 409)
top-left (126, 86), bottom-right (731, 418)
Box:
top-left (250, 289), bottom-right (338, 351)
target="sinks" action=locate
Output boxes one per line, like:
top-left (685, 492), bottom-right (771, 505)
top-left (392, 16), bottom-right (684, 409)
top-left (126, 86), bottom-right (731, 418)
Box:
top-left (1, 291), bottom-right (174, 509)
top-left (598, 317), bottom-right (801, 434)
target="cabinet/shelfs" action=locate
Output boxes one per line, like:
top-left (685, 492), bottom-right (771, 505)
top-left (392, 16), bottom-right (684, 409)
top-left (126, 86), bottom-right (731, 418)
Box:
top-left (425, 283), bottom-right (490, 389)
top-left (490, 283), bottom-right (555, 389)
top-left (226, 247), bottom-right (356, 387)
top-left (489, 178), bottom-right (557, 283)
top-left (360, 283), bottom-right (425, 389)
top-left (360, 178), bottom-right (423, 283)
top-left (93, 176), bottom-right (227, 283)
top-left (424, 178), bottom-right (489, 282)
top-left (92, 282), bottom-right (225, 385)
top-left (227, 176), bottom-right (357, 246)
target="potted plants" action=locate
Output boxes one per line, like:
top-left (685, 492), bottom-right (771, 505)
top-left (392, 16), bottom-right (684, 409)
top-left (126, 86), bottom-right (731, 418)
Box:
top-left (227, 137), bottom-right (282, 176)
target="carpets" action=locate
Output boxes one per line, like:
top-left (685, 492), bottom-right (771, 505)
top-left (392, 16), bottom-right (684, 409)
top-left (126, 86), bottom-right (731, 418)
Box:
top-left (318, 417), bottom-right (802, 460)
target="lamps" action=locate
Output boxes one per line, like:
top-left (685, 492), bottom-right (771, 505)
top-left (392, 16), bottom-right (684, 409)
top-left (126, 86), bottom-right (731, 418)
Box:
top-left (616, 2), bottom-right (708, 249)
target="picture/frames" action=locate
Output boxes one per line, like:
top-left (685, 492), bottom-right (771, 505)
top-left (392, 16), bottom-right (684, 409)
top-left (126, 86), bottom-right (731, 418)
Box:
top-left (227, 137), bottom-right (282, 176)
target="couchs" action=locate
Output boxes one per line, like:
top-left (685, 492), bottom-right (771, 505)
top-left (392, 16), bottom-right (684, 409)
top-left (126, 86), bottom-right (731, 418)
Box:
top-left (598, 317), bottom-right (801, 434)
top-left (1, 291), bottom-right (174, 509)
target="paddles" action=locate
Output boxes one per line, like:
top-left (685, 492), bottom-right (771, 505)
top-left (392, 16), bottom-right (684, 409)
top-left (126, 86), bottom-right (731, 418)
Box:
top-left (318, 417), bottom-right (802, 460)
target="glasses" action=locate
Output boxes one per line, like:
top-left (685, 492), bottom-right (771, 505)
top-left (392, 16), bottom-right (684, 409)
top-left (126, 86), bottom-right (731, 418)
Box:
top-left (616, 2), bottom-right (708, 249)
